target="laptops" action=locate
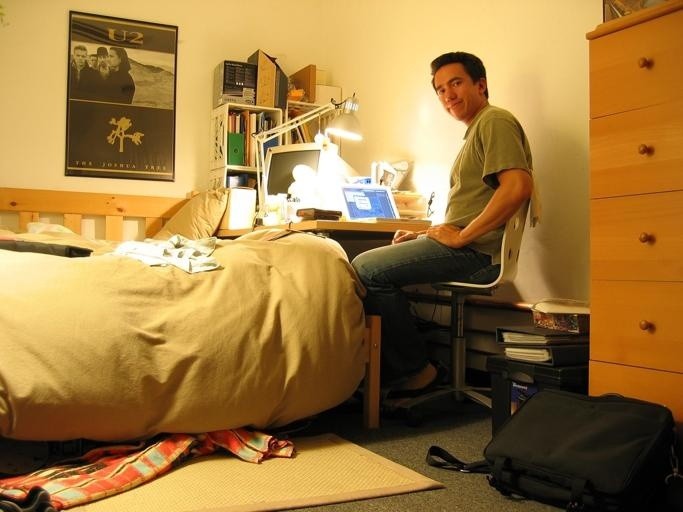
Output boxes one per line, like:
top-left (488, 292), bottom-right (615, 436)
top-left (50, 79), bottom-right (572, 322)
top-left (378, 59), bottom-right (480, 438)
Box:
top-left (339, 184), bottom-right (431, 223)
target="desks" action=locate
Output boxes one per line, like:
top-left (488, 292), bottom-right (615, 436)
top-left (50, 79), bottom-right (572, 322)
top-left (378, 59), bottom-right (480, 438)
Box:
top-left (218, 219), bottom-right (433, 236)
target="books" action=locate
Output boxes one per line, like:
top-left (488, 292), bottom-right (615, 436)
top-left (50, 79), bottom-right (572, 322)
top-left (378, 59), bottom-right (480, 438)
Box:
top-left (227, 174), bottom-right (257, 188)
top-left (283, 117), bottom-right (311, 146)
top-left (496, 325), bottom-right (589, 366)
top-left (297, 208), bottom-right (342, 220)
top-left (228, 111), bottom-right (279, 167)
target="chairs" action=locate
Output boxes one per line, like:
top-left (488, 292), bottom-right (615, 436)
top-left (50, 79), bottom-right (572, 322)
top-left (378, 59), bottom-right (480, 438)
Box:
top-left (380, 196), bottom-right (530, 426)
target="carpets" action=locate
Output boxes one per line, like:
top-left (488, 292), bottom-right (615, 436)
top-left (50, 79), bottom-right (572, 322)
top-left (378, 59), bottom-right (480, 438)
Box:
top-left (56, 432), bottom-right (447, 512)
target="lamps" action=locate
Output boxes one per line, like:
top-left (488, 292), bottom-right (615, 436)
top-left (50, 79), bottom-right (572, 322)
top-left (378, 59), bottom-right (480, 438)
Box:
top-left (252, 92), bottom-right (363, 219)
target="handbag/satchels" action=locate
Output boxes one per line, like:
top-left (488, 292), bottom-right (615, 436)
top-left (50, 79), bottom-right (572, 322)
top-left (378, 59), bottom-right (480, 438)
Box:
top-left (483, 386), bottom-right (678, 511)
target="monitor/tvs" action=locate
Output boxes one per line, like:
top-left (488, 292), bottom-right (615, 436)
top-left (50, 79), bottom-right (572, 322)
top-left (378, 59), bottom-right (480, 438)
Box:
top-left (261, 143), bottom-right (361, 226)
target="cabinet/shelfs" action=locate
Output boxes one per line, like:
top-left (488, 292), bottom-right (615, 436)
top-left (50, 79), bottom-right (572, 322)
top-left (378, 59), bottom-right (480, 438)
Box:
top-left (585, 2), bottom-right (683, 428)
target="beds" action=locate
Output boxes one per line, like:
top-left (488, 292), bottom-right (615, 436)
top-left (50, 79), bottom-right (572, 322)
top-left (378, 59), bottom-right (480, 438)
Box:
top-left (0, 188), bottom-right (364, 442)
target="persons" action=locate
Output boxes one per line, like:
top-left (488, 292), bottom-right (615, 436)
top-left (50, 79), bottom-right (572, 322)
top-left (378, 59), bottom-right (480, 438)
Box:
top-left (351, 52), bottom-right (533, 400)
top-left (70, 46), bottom-right (135, 104)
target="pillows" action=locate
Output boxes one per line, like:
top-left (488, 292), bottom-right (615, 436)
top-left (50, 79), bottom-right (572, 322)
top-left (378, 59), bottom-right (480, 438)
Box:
top-left (152, 189), bottom-right (229, 242)
